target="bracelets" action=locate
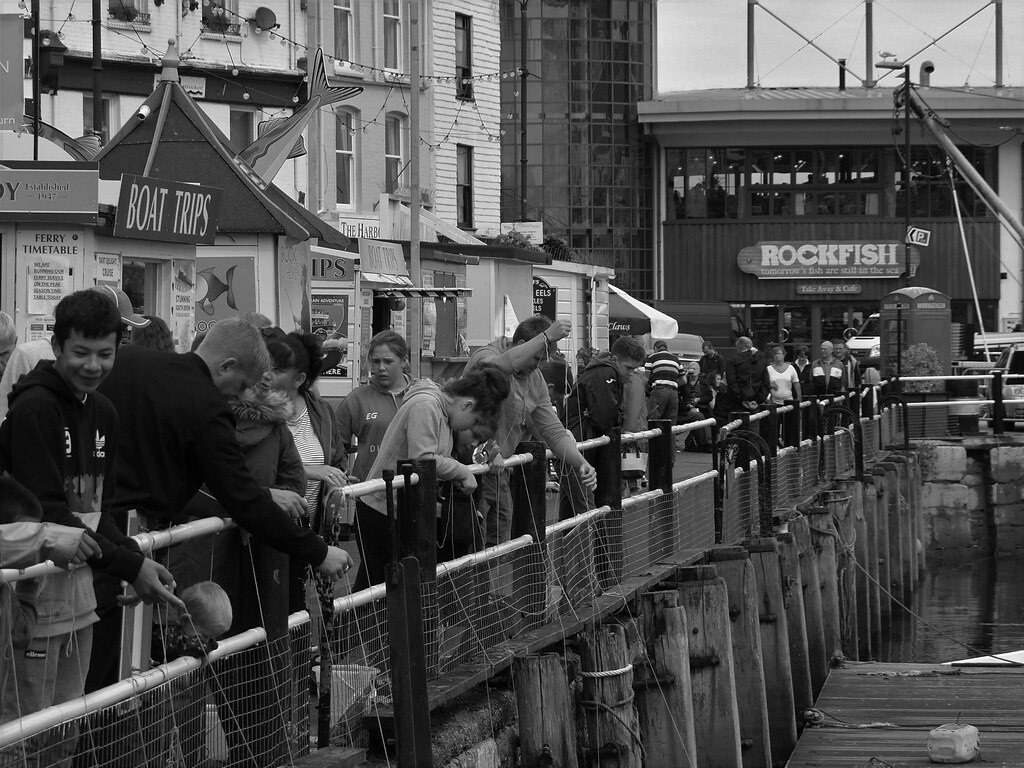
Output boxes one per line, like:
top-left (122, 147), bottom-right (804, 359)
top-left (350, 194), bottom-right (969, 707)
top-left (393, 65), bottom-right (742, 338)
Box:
top-left (498, 450), bottom-right (504, 458)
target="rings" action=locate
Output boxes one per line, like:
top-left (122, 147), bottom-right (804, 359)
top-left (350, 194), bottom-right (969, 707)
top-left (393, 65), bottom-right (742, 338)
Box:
top-left (330, 473), bottom-right (333, 478)
top-left (344, 565), bottom-right (349, 572)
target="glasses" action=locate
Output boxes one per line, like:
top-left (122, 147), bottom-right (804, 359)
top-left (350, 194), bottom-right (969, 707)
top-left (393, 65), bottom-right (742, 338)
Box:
top-left (262, 327), bottom-right (281, 338)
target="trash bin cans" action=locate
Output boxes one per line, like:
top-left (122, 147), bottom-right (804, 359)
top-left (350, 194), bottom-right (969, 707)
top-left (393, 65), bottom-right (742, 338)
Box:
top-left (944, 375), bottom-right (979, 436)
top-left (430, 356), bottom-right (471, 385)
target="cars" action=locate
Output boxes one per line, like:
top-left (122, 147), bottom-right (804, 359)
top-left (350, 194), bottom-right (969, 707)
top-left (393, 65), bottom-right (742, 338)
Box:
top-left (986, 340), bottom-right (1024, 430)
top-left (643, 332), bottom-right (709, 372)
top-left (842, 335), bottom-right (883, 359)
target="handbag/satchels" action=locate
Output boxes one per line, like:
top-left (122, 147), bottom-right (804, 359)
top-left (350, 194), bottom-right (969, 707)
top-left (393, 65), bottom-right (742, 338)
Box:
top-left (739, 385), bottom-right (754, 398)
top-left (621, 441), bottom-right (648, 479)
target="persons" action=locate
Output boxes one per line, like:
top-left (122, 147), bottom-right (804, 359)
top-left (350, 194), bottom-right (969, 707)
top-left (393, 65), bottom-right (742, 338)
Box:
top-left (685, 174), bottom-right (926, 213)
top-left (0, 284), bottom-right (862, 768)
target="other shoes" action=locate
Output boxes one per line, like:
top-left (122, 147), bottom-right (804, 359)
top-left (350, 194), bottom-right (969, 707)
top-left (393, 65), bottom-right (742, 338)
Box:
top-left (700, 445), bottom-right (712, 453)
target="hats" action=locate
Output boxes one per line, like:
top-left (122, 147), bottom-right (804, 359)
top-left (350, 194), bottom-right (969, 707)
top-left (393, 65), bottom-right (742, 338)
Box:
top-left (88, 284), bottom-right (151, 329)
